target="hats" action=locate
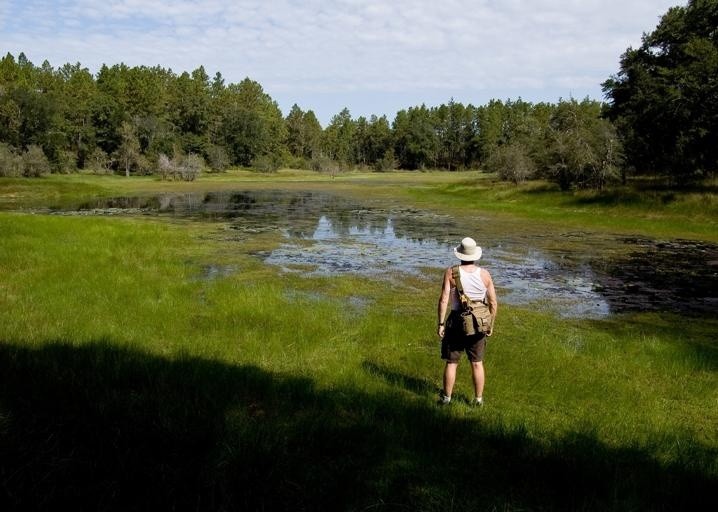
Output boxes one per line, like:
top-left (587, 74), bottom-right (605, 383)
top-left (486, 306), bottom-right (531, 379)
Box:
top-left (454, 237), bottom-right (482, 262)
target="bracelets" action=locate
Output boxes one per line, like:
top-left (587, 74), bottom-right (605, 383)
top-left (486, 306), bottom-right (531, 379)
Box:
top-left (437, 323), bottom-right (444, 325)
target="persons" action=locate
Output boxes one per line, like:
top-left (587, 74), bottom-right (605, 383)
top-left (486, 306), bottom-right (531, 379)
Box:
top-left (438, 238), bottom-right (499, 405)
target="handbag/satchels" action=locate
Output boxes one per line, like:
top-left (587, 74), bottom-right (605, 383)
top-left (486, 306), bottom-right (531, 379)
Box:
top-left (460, 301), bottom-right (492, 336)
